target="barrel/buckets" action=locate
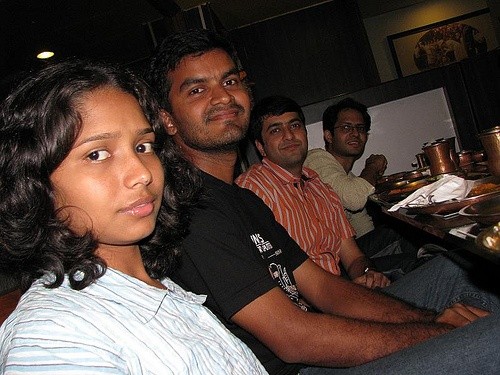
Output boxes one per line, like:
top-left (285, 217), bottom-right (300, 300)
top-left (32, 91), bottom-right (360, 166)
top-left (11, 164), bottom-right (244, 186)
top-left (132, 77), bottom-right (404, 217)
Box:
top-left (422, 137), bottom-right (458, 175)
top-left (479, 127), bottom-right (500, 176)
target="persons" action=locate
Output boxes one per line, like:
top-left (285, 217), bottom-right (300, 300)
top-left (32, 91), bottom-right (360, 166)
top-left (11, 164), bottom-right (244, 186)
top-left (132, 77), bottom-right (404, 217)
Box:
top-left (235, 95), bottom-right (500, 314)
top-left (303, 98), bottom-right (414, 257)
top-left (0, 57), bottom-right (269, 375)
top-left (140, 28), bottom-right (500, 375)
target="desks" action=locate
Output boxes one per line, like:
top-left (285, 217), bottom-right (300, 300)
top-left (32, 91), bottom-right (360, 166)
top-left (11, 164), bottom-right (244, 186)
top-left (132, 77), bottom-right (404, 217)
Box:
top-left (367, 163), bottom-right (500, 269)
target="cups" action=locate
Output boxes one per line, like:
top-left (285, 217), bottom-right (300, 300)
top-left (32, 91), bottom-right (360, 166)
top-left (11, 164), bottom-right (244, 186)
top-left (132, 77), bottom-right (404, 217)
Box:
top-left (415, 153), bottom-right (427, 166)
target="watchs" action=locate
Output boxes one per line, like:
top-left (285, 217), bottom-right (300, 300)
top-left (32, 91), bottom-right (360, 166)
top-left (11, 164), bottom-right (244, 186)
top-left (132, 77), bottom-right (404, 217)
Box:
top-left (362, 266), bottom-right (381, 273)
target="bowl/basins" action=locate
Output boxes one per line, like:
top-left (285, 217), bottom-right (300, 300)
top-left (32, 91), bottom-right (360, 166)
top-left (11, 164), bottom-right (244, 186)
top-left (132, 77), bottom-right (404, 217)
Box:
top-left (459, 198), bottom-right (500, 224)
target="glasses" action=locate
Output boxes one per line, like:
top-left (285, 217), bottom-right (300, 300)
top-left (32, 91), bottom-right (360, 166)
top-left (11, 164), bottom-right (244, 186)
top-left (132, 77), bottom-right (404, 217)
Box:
top-left (334, 125), bottom-right (366, 132)
